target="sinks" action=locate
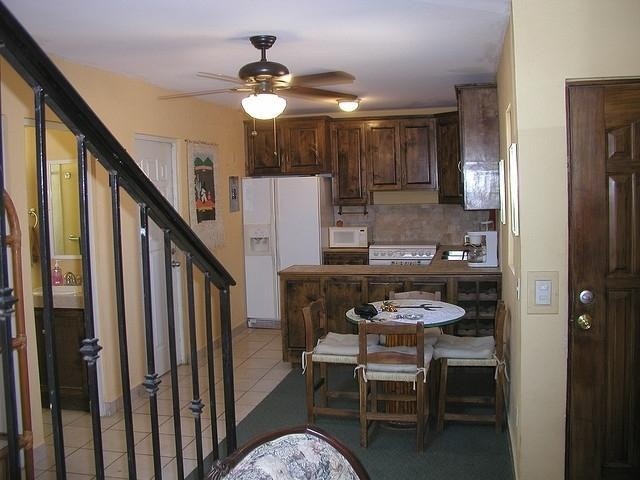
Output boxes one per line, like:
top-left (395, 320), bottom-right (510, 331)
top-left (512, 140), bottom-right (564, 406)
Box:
top-left (32, 283), bottom-right (83, 310)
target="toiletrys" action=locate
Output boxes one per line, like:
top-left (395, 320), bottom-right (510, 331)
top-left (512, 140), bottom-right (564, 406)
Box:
top-left (51, 260), bottom-right (64, 286)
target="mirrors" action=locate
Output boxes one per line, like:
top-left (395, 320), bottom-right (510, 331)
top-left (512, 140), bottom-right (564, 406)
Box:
top-left (47, 160), bottom-right (84, 260)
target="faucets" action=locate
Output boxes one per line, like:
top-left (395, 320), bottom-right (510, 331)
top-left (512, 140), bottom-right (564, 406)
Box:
top-left (63, 271), bottom-right (77, 286)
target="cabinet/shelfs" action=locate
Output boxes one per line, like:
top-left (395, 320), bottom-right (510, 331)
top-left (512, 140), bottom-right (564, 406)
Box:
top-left (330, 121), bottom-right (374, 206)
top-left (365, 117), bottom-right (439, 191)
top-left (285, 274), bottom-right (362, 349)
top-left (324, 252), bottom-right (369, 265)
top-left (33, 307), bottom-right (90, 414)
top-left (457, 88), bottom-right (500, 211)
top-left (362, 274), bottom-right (448, 305)
top-left (244, 119), bottom-right (331, 176)
top-left (437, 115), bottom-right (464, 205)
top-left (448, 275), bottom-right (502, 336)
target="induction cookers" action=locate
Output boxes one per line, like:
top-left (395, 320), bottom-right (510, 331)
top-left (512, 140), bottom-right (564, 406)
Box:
top-left (368, 240), bottom-right (439, 259)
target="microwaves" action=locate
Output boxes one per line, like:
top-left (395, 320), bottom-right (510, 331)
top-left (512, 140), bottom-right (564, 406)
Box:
top-left (329, 224), bottom-right (371, 250)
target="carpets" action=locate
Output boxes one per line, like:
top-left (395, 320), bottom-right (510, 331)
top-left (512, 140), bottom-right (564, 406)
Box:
top-left (185, 363), bottom-right (517, 480)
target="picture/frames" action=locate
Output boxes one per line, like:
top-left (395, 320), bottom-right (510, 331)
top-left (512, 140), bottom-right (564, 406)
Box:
top-left (229, 176), bottom-right (239, 212)
top-left (507, 143), bottom-right (520, 237)
top-left (498, 159), bottom-right (506, 225)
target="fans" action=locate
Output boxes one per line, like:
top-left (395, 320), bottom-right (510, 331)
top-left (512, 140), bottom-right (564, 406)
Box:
top-left (156, 35), bottom-right (357, 104)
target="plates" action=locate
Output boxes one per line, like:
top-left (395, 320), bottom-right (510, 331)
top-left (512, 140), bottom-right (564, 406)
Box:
top-left (405, 314), bottom-right (423, 320)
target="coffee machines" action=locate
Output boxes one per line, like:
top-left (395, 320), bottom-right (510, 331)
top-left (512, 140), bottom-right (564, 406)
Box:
top-left (465, 230), bottom-right (501, 268)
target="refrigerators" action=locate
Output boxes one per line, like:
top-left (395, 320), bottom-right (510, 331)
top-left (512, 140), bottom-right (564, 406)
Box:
top-left (238, 176), bottom-right (335, 332)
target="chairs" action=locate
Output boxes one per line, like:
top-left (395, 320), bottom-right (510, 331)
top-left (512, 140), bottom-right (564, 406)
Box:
top-left (202, 424), bottom-right (371, 480)
top-left (301, 290), bottom-right (507, 458)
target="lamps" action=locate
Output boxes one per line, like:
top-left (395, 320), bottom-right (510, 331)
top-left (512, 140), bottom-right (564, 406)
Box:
top-left (241, 93), bottom-right (287, 120)
top-left (336, 97), bottom-right (361, 112)
